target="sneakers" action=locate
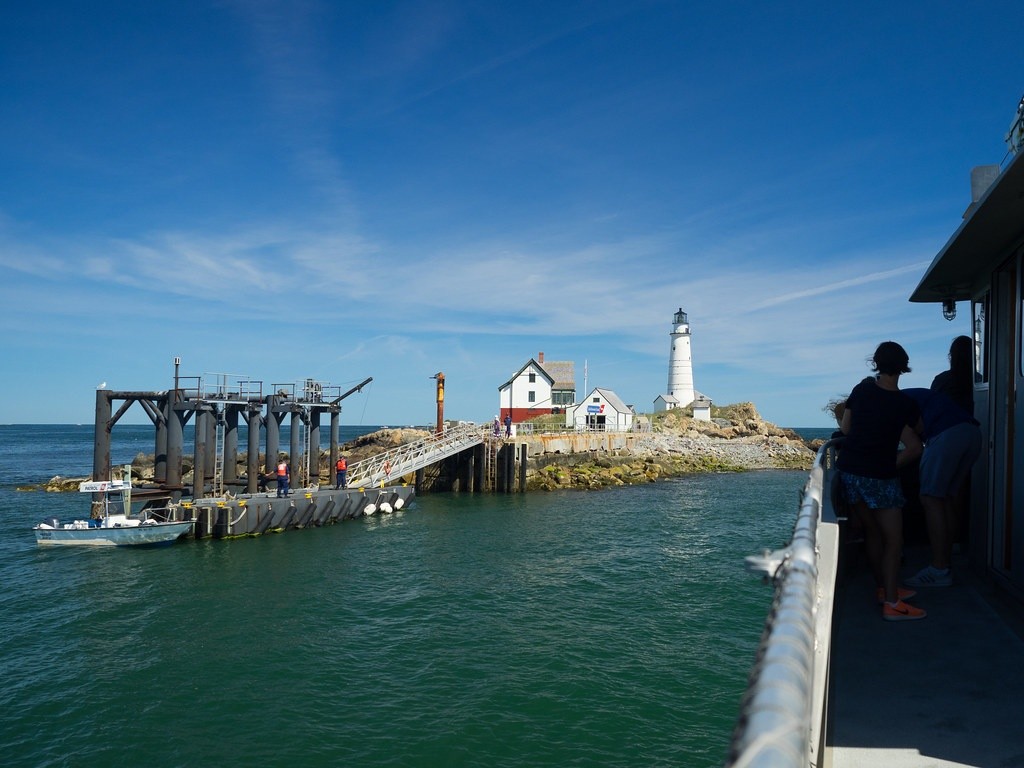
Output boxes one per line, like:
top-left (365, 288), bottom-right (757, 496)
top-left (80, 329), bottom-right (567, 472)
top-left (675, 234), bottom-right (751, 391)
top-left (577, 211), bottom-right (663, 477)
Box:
top-left (879, 584), bottom-right (915, 601)
top-left (882, 603), bottom-right (930, 621)
top-left (904, 567), bottom-right (953, 588)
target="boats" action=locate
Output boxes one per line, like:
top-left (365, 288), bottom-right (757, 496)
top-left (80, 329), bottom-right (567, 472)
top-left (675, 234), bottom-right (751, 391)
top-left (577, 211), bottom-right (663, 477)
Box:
top-left (380, 425), bottom-right (389, 428)
top-left (31, 521), bottom-right (198, 547)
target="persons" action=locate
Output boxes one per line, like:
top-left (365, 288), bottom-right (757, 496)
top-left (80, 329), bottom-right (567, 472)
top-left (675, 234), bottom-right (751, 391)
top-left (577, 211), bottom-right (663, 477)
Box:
top-left (265, 459), bottom-right (292, 498)
top-left (334, 454), bottom-right (348, 489)
top-left (834, 335), bottom-right (981, 621)
top-left (505, 412), bottom-right (513, 438)
top-left (495, 416), bottom-right (501, 438)
